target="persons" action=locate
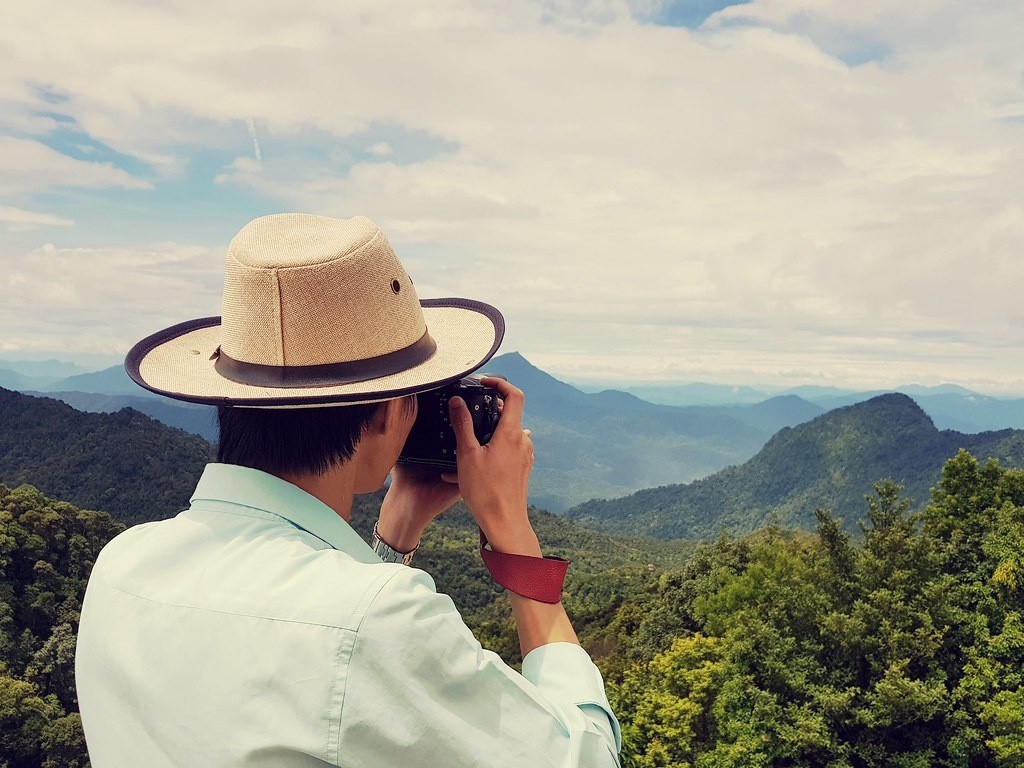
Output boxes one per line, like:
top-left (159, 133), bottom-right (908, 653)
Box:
top-left (74, 212), bottom-right (623, 768)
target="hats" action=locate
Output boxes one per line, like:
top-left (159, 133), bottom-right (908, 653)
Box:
top-left (125, 213), bottom-right (505, 408)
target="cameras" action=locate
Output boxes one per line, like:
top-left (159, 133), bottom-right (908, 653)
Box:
top-left (395, 372), bottom-right (507, 470)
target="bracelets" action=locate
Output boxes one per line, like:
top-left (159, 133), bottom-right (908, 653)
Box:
top-left (371, 520), bottom-right (420, 566)
